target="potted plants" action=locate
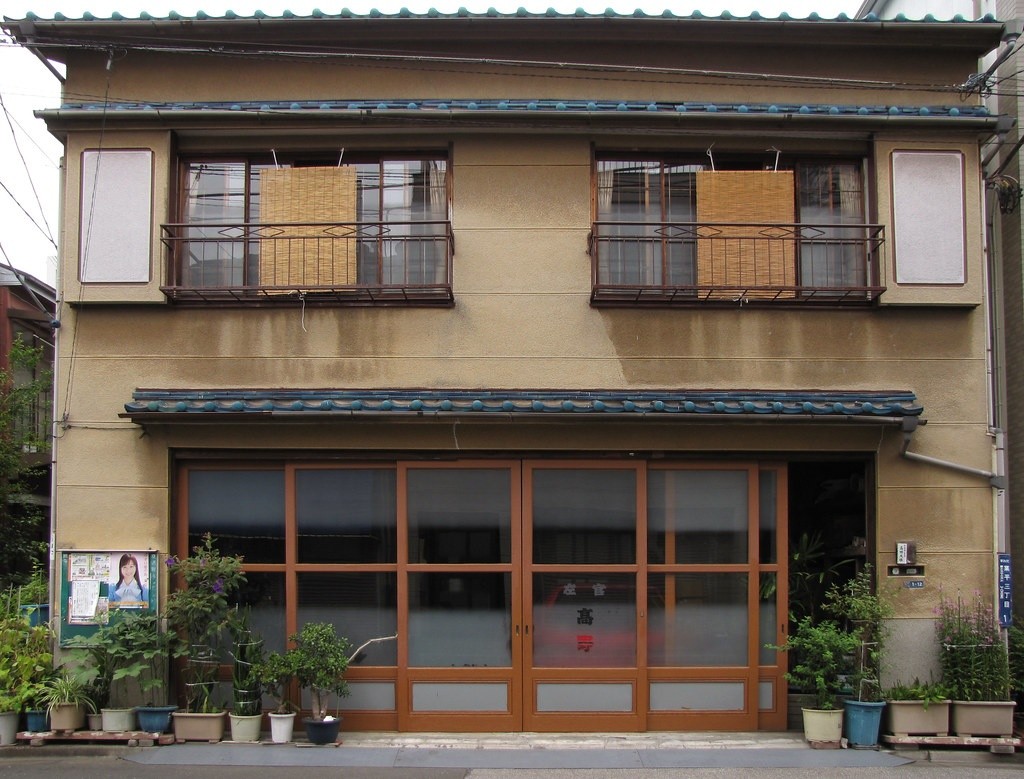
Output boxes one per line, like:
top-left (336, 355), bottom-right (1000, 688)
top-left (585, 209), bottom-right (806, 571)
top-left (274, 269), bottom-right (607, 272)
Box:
top-left (0, 590), bottom-right (181, 747)
top-left (764, 562), bottom-right (953, 744)
top-left (226, 601), bottom-right (353, 743)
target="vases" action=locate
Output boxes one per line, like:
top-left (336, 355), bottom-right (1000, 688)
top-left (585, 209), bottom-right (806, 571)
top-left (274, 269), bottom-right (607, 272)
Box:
top-left (951, 697), bottom-right (1017, 739)
top-left (174, 711), bottom-right (225, 743)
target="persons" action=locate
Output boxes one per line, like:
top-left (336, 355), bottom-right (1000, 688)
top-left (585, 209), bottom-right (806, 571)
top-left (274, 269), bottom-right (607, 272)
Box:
top-left (106, 554), bottom-right (148, 608)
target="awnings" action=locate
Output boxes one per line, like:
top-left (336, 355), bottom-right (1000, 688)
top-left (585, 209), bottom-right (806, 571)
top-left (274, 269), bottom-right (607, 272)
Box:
top-left (37, 94), bottom-right (1001, 144)
top-left (117, 388), bottom-right (929, 440)
top-left (0, 9), bottom-right (1009, 68)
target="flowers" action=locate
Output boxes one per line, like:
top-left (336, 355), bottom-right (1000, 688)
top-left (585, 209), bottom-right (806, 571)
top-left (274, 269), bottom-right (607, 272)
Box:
top-left (164, 529), bottom-right (248, 713)
top-left (931, 577), bottom-right (1018, 701)
